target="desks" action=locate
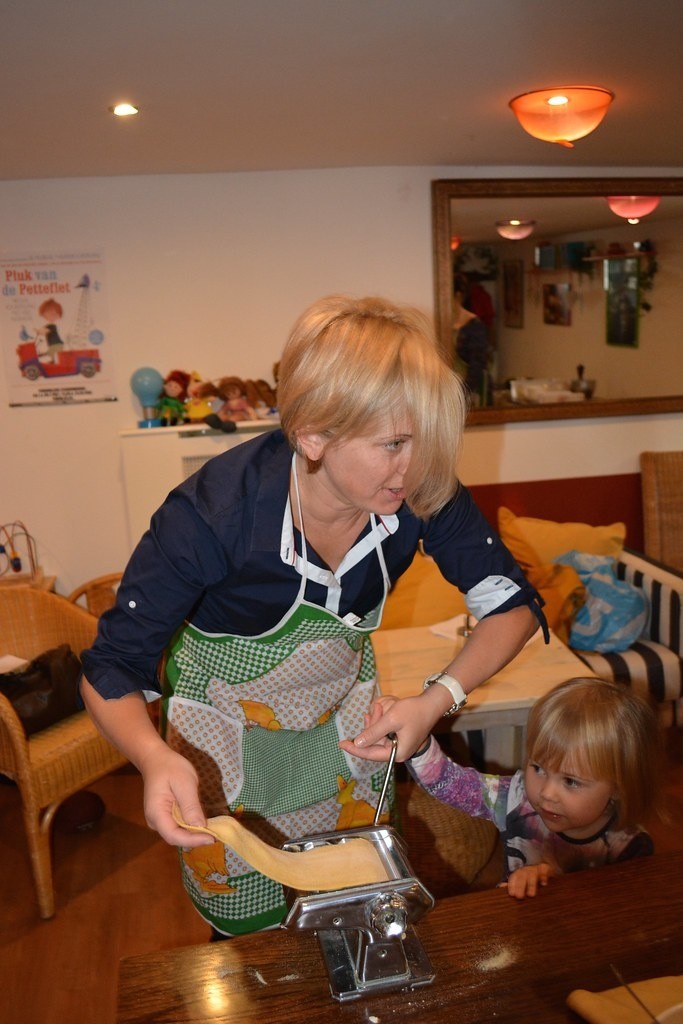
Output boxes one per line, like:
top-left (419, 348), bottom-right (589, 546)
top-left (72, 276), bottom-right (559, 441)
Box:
top-left (369, 621), bottom-right (599, 771)
top-left (121, 419), bottom-right (279, 559)
top-left (120, 849), bottom-right (683, 1024)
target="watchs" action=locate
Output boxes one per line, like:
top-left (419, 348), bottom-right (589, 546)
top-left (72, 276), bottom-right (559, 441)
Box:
top-left (423, 672), bottom-right (468, 718)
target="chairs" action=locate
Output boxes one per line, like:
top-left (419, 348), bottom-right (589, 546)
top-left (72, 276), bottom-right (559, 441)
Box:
top-left (0, 585), bottom-right (160, 918)
top-left (392, 772), bottom-right (512, 899)
top-left (641, 451), bottom-right (683, 574)
top-left (68, 573), bottom-right (123, 623)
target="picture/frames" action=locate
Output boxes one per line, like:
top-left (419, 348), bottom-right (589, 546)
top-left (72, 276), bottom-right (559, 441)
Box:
top-left (606, 261), bottom-right (640, 347)
top-left (541, 285), bottom-right (570, 326)
top-left (502, 258), bottom-right (523, 329)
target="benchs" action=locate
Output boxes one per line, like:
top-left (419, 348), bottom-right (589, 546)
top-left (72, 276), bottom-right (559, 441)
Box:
top-left (370, 550), bottom-right (683, 772)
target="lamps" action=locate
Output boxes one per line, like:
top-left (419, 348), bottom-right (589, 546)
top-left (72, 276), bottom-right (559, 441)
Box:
top-left (496, 219), bottom-right (537, 241)
top-left (605, 196), bottom-right (660, 224)
top-left (507, 85), bottom-right (616, 149)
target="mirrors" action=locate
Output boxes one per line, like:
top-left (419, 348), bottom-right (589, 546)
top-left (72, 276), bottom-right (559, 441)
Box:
top-left (428, 178), bottom-right (683, 422)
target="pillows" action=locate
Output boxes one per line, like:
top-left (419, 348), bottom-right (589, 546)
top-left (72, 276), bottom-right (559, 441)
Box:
top-left (380, 550), bottom-right (472, 629)
top-left (498, 507), bottom-right (626, 655)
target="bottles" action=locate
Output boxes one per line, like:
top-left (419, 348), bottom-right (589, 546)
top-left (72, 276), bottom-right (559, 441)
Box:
top-left (455, 626), bottom-right (475, 655)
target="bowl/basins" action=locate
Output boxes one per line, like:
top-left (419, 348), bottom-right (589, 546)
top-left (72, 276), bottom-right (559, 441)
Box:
top-left (570, 379), bottom-right (595, 400)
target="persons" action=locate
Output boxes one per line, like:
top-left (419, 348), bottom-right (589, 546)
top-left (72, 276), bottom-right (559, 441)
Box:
top-left (153, 360), bottom-right (281, 433)
top-left (78, 296), bottom-right (549, 943)
top-left (453, 271), bottom-right (489, 407)
top-left (368, 677), bottom-right (659, 900)
